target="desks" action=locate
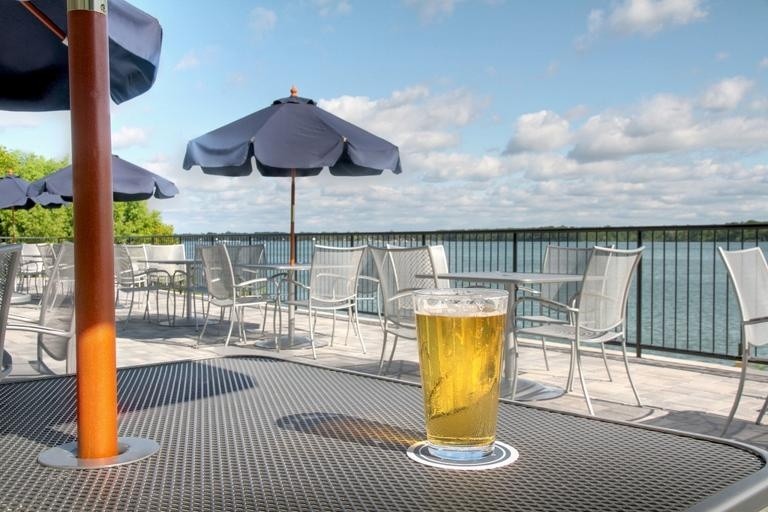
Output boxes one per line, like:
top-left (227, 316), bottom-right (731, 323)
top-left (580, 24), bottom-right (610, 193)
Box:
top-left (1, 356), bottom-right (768, 511)
top-left (411, 270), bottom-right (610, 404)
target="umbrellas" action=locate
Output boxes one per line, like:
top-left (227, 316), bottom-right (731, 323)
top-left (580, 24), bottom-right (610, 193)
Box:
top-left (1, 170), bottom-right (64, 243)
top-left (27, 154), bottom-right (179, 204)
top-left (182, 86), bottom-right (403, 265)
top-left (0, 1), bottom-right (163, 112)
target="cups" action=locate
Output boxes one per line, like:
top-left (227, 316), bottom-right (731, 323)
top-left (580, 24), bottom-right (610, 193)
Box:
top-left (413, 287), bottom-right (512, 462)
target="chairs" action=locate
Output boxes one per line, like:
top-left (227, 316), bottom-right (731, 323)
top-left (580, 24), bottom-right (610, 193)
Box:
top-left (112, 238), bottom-right (389, 360)
top-left (509, 239), bottom-right (645, 417)
top-left (718, 247), bottom-right (768, 434)
top-left (0, 239), bottom-right (75, 378)
top-left (367, 242), bottom-right (451, 377)
